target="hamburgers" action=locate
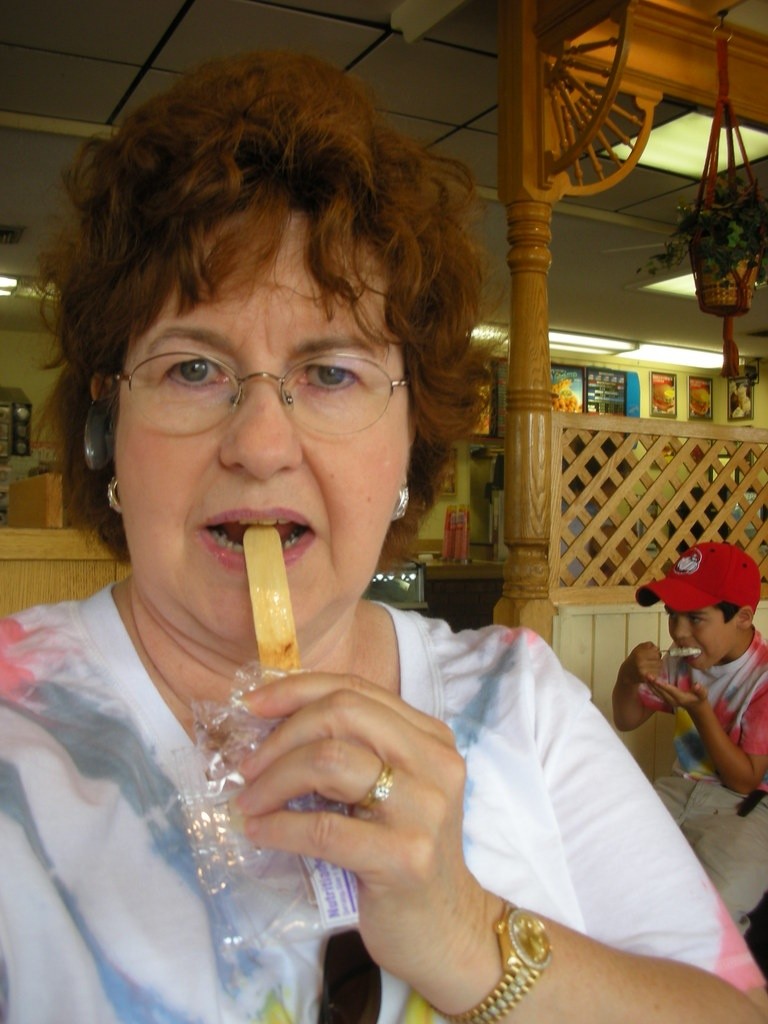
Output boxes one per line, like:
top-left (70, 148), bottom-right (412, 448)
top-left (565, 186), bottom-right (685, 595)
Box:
top-left (689, 388), bottom-right (709, 415)
top-left (653, 383), bottom-right (675, 410)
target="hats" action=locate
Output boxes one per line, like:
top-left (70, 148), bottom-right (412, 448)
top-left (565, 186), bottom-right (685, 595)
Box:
top-left (635, 541), bottom-right (761, 615)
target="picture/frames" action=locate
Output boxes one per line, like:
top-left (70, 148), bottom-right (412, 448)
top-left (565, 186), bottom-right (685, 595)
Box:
top-left (728, 377), bottom-right (754, 422)
top-left (686, 375), bottom-right (714, 421)
top-left (650, 371), bottom-right (678, 419)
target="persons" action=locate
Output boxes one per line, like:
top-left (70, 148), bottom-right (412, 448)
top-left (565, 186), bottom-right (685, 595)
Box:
top-left (611, 542), bottom-right (768, 936)
top-left (0, 47), bottom-right (768, 1024)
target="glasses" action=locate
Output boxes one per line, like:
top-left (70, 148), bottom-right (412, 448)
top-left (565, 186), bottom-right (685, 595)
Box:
top-left (115, 351), bottom-right (409, 438)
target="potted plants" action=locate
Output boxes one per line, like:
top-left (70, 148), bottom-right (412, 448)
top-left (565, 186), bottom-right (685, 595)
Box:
top-left (635, 174), bottom-right (768, 317)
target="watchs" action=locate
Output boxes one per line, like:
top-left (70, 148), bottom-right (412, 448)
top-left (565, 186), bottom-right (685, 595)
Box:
top-left (427, 896), bottom-right (556, 1024)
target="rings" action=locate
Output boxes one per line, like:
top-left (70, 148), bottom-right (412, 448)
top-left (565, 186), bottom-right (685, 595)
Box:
top-left (356, 759), bottom-right (394, 811)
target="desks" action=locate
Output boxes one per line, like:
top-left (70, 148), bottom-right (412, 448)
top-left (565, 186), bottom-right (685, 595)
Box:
top-left (423, 556), bottom-right (504, 630)
